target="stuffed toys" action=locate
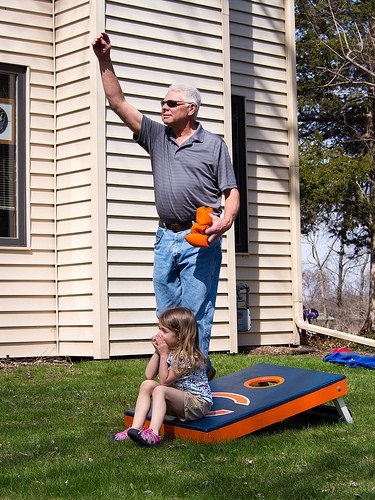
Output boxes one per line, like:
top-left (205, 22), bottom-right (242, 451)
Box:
top-left (185, 206), bottom-right (215, 247)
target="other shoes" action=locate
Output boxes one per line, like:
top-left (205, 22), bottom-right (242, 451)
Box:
top-left (207, 367), bottom-right (217, 382)
top-left (111, 427), bottom-right (137, 440)
top-left (128, 428), bottom-right (163, 447)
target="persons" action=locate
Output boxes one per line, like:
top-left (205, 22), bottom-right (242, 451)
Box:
top-left (92, 33), bottom-right (240, 382)
top-left (111, 307), bottom-right (214, 446)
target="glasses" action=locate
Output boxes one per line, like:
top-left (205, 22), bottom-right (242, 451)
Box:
top-left (161, 100), bottom-right (187, 108)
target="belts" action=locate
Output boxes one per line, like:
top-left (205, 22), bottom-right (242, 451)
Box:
top-left (159, 215), bottom-right (220, 233)
